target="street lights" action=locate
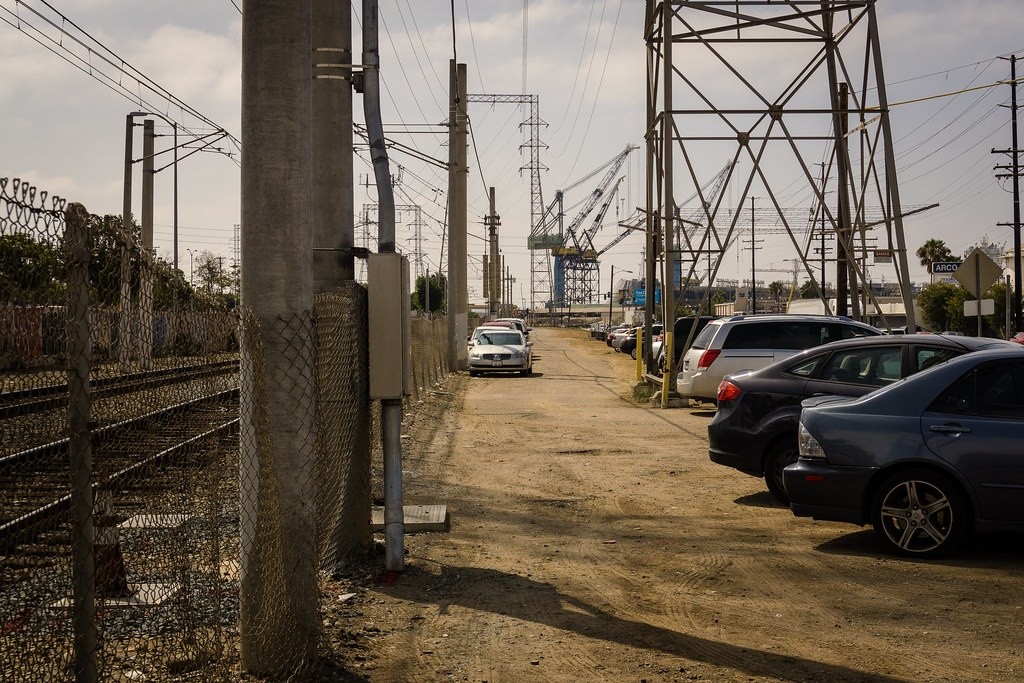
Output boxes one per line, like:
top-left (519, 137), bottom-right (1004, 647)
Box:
top-left (609, 264), bottom-right (634, 334)
top-left (782, 258), bottom-right (826, 297)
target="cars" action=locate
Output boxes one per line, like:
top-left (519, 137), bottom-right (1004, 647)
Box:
top-left (706, 334), bottom-right (1024, 509)
top-left (467, 332), bottom-right (530, 377)
top-left (1009, 332), bottom-right (1023, 346)
top-left (591, 315), bottom-right (721, 367)
top-left (782, 347), bottom-right (1024, 560)
top-left (465, 317), bottom-right (533, 354)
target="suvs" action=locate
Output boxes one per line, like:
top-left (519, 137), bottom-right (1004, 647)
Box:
top-left (676, 313), bottom-right (887, 409)
